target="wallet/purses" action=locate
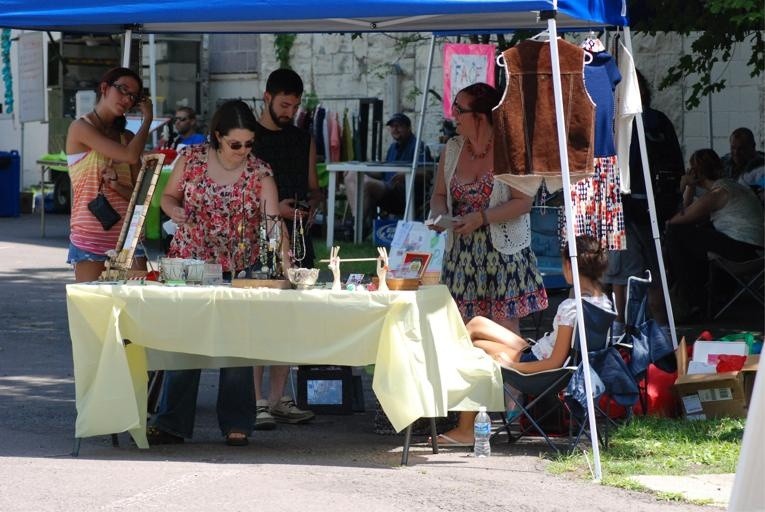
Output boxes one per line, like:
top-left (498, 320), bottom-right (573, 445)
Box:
top-left (88, 195), bottom-right (121, 231)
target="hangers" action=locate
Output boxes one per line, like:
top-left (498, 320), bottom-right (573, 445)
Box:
top-left (585, 28), bottom-right (598, 41)
top-left (613, 26), bottom-right (634, 59)
top-left (496, 13), bottom-right (593, 67)
top-left (232, 95), bottom-right (362, 122)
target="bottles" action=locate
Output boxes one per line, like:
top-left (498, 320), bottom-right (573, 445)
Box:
top-left (702, 330), bottom-right (713, 341)
top-left (506, 389), bottom-right (522, 422)
top-left (472, 406), bottom-right (492, 456)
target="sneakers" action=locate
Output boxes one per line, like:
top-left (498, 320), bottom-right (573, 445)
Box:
top-left (254, 398), bottom-right (276, 430)
top-left (270, 395), bottom-right (316, 424)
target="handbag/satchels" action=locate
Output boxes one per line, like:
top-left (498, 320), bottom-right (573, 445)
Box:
top-left (656, 170), bottom-right (681, 196)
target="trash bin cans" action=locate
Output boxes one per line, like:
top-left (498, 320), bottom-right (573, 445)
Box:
top-left (0, 150), bottom-right (20, 217)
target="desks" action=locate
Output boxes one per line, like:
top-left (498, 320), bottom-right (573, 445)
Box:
top-left (326, 161), bottom-right (439, 250)
top-left (65, 284), bottom-right (439, 465)
top-left (36, 159), bottom-right (69, 238)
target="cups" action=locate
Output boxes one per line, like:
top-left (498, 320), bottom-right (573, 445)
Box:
top-left (421, 271), bottom-right (441, 285)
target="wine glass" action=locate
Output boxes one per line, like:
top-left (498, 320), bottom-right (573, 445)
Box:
top-left (287, 267), bottom-right (320, 291)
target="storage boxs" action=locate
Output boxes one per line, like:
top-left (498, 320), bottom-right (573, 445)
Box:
top-left (372, 218), bottom-right (399, 248)
top-left (673, 336), bottom-right (760, 424)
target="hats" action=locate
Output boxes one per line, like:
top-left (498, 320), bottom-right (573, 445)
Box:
top-left (385, 113), bottom-right (410, 126)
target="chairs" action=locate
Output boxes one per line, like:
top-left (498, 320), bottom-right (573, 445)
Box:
top-left (486, 292), bottom-right (619, 459)
top-left (705, 252), bottom-right (765, 324)
top-left (595, 269), bottom-right (654, 435)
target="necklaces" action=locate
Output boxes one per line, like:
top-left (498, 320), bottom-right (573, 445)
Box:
top-left (464, 135), bottom-right (497, 158)
top-left (93, 108), bottom-right (107, 129)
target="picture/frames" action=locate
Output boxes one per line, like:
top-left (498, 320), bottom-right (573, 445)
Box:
top-left (396, 252), bottom-right (432, 278)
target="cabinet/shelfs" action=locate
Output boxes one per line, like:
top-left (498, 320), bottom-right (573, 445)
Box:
top-left (48, 38), bottom-right (140, 183)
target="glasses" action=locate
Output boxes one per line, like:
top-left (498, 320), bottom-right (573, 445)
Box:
top-left (453, 102), bottom-right (484, 114)
top-left (106, 81), bottom-right (138, 103)
top-left (173, 115), bottom-right (192, 122)
top-left (220, 134), bottom-right (255, 149)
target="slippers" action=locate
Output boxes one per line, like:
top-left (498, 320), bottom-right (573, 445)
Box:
top-left (428, 434), bottom-right (474, 447)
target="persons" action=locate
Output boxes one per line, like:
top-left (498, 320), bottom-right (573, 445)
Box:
top-left (253, 67), bottom-right (323, 429)
top-left (134, 101), bottom-right (294, 446)
top-left (67, 65), bottom-right (154, 282)
top-left (427, 232), bottom-right (614, 449)
top-left (162, 105), bottom-right (208, 147)
top-left (342, 112), bottom-right (434, 238)
top-left (602, 69), bottom-right (685, 332)
top-left (426, 81), bottom-right (550, 446)
top-left (664, 146), bottom-right (763, 317)
top-left (719, 127), bottom-right (764, 191)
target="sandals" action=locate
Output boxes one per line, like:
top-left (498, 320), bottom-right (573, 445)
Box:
top-left (130, 426), bottom-right (185, 444)
top-left (226, 429), bottom-right (250, 446)
